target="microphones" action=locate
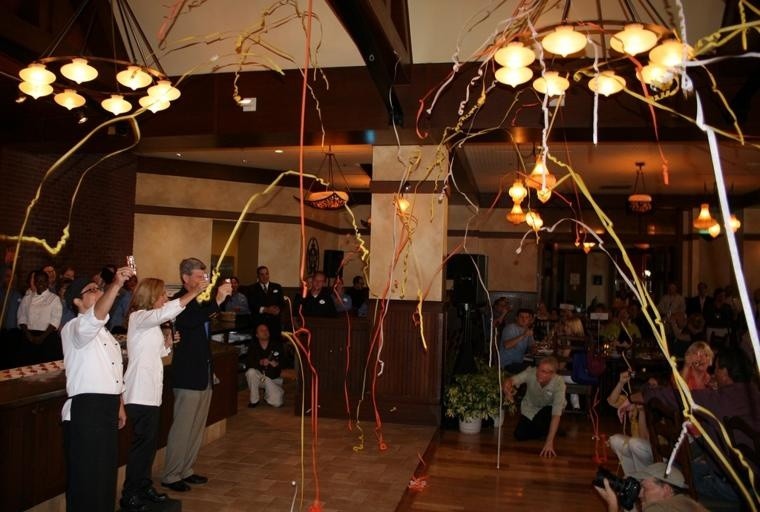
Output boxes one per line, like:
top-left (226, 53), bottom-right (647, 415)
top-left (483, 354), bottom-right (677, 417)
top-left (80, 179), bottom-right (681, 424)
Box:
top-left (170, 317), bottom-right (178, 349)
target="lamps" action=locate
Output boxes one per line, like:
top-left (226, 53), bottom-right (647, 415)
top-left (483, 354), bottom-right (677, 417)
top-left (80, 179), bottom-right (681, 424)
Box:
top-left (15, 1), bottom-right (185, 118)
top-left (488, 0), bottom-right (690, 101)
top-left (507, 142), bottom-right (747, 256)
top-left (394, 180), bottom-right (413, 212)
top-left (294, 150), bottom-right (364, 212)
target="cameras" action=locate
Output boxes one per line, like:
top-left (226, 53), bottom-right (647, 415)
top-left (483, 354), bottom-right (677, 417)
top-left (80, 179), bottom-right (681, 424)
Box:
top-left (592, 466), bottom-right (640, 511)
top-left (629, 370), bottom-right (639, 379)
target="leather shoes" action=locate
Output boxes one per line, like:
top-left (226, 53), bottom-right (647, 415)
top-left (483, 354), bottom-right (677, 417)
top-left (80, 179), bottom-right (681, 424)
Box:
top-left (120, 492), bottom-right (145, 511)
top-left (247, 401), bottom-right (261, 409)
top-left (141, 480), bottom-right (166, 502)
top-left (186, 475), bottom-right (208, 484)
top-left (163, 479), bottom-right (193, 492)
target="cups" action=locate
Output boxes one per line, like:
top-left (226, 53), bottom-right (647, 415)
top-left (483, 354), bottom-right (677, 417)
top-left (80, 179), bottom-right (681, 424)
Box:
top-left (127, 255), bottom-right (137, 275)
top-left (225, 278), bottom-right (232, 284)
top-left (202, 272), bottom-right (210, 281)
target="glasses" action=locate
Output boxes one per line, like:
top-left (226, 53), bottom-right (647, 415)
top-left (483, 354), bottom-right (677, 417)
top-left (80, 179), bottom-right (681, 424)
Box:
top-left (81, 286), bottom-right (103, 297)
top-left (46, 269), bottom-right (56, 275)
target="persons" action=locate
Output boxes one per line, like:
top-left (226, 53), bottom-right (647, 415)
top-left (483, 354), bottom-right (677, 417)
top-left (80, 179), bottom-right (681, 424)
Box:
top-left (119, 277), bottom-right (209, 512)
top-left (503, 357), bottom-right (568, 459)
top-left (444, 280), bottom-right (759, 434)
top-left (1, 263), bottom-right (369, 373)
top-left (159, 258), bottom-right (233, 492)
top-left (594, 462), bottom-right (714, 512)
top-left (59, 264), bottom-right (135, 511)
top-left (606, 369), bottom-right (669, 476)
top-left (242, 324), bottom-right (286, 408)
top-left (688, 344), bottom-right (759, 481)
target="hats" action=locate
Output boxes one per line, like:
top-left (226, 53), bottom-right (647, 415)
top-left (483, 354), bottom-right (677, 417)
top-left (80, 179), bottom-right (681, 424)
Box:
top-left (628, 462), bottom-right (690, 489)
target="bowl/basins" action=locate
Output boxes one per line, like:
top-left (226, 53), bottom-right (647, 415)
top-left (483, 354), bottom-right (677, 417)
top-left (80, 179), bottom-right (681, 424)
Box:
top-left (544, 349), bottom-right (554, 355)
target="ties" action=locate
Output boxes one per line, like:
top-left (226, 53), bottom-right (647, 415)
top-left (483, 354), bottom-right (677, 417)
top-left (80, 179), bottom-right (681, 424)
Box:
top-left (263, 283), bottom-right (268, 293)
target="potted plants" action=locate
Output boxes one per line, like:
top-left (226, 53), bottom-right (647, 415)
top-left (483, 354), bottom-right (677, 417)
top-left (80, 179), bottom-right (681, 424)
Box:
top-left (446, 373), bottom-right (494, 435)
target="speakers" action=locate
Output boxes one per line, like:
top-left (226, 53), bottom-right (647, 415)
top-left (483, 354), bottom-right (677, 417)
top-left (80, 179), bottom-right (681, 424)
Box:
top-left (324, 249), bottom-right (344, 278)
